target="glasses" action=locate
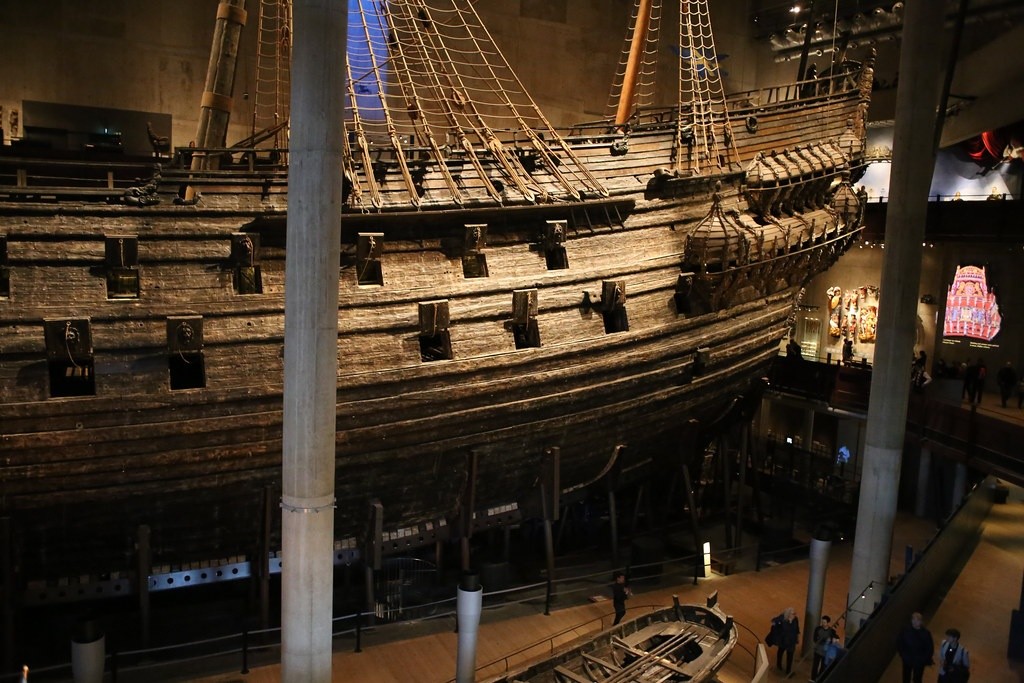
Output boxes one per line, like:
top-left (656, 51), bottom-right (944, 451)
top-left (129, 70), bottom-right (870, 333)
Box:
top-left (792, 612), bottom-right (796, 615)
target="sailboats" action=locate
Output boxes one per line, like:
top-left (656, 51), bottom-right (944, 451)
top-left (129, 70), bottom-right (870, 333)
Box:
top-left (0, 0), bottom-right (875, 563)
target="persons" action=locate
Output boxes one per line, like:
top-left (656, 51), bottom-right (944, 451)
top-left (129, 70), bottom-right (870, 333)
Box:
top-left (765, 607), bottom-right (801, 679)
top-left (896, 612), bottom-right (934, 683)
top-left (807, 615), bottom-right (840, 683)
top-left (612, 573), bottom-right (633, 626)
top-left (936, 629), bottom-right (970, 683)
top-left (911, 350), bottom-right (1024, 408)
top-left (843, 338), bottom-right (854, 368)
top-left (785, 338), bottom-right (804, 359)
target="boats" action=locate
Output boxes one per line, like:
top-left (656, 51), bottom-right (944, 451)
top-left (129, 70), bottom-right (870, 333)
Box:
top-left (488, 592), bottom-right (739, 683)
